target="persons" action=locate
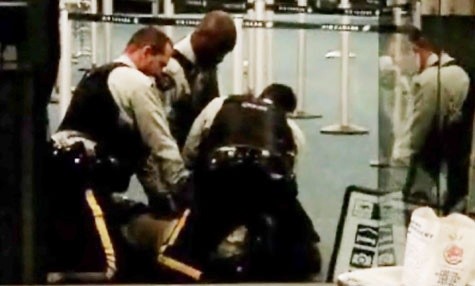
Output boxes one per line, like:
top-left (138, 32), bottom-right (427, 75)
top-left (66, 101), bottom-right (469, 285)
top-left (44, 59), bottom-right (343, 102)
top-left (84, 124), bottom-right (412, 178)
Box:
top-left (38, 25), bottom-right (190, 286)
top-left (382, 22), bottom-right (475, 269)
top-left (151, 81), bottom-right (305, 285)
top-left (137, 9), bottom-right (238, 213)
top-left (113, 198), bottom-right (249, 267)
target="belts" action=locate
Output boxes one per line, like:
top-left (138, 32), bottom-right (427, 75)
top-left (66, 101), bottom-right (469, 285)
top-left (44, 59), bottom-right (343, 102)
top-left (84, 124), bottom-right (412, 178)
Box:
top-left (211, 147), bottom-right (270, 167)
